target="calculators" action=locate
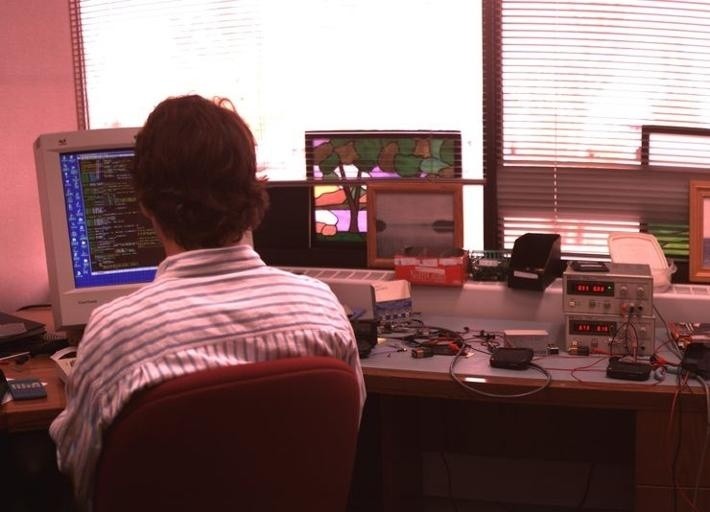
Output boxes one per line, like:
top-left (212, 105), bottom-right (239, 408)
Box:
top-left (7, 379), bottom-right (46, 400)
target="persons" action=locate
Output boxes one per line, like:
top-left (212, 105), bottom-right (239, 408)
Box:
top-left (50, 94), bottom-right (367, 512)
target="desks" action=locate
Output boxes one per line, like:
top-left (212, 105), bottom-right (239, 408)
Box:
top-left (0, 308), bottom-right (710, 512)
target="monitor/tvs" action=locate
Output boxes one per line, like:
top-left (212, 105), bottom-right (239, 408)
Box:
top-left (33, 127), bottom-right (254, 345)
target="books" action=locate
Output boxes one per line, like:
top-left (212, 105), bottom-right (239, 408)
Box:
top-left (668, 319), bottom-right (710, 349)
top-left (0, 310), bottom-right (49, 345)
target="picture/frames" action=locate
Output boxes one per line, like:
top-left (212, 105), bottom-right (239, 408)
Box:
top-left (305, 131), bottom-right (462, 248)
top-left (688, 180), bottom-right (710, 282)
top-left (638, 124), bottom-right (710, 285)
top-left (366, 183), bottom-right (463, 270)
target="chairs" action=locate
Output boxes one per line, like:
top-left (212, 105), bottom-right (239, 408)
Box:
top-left (89, 356), bottom-right (361, 512)
